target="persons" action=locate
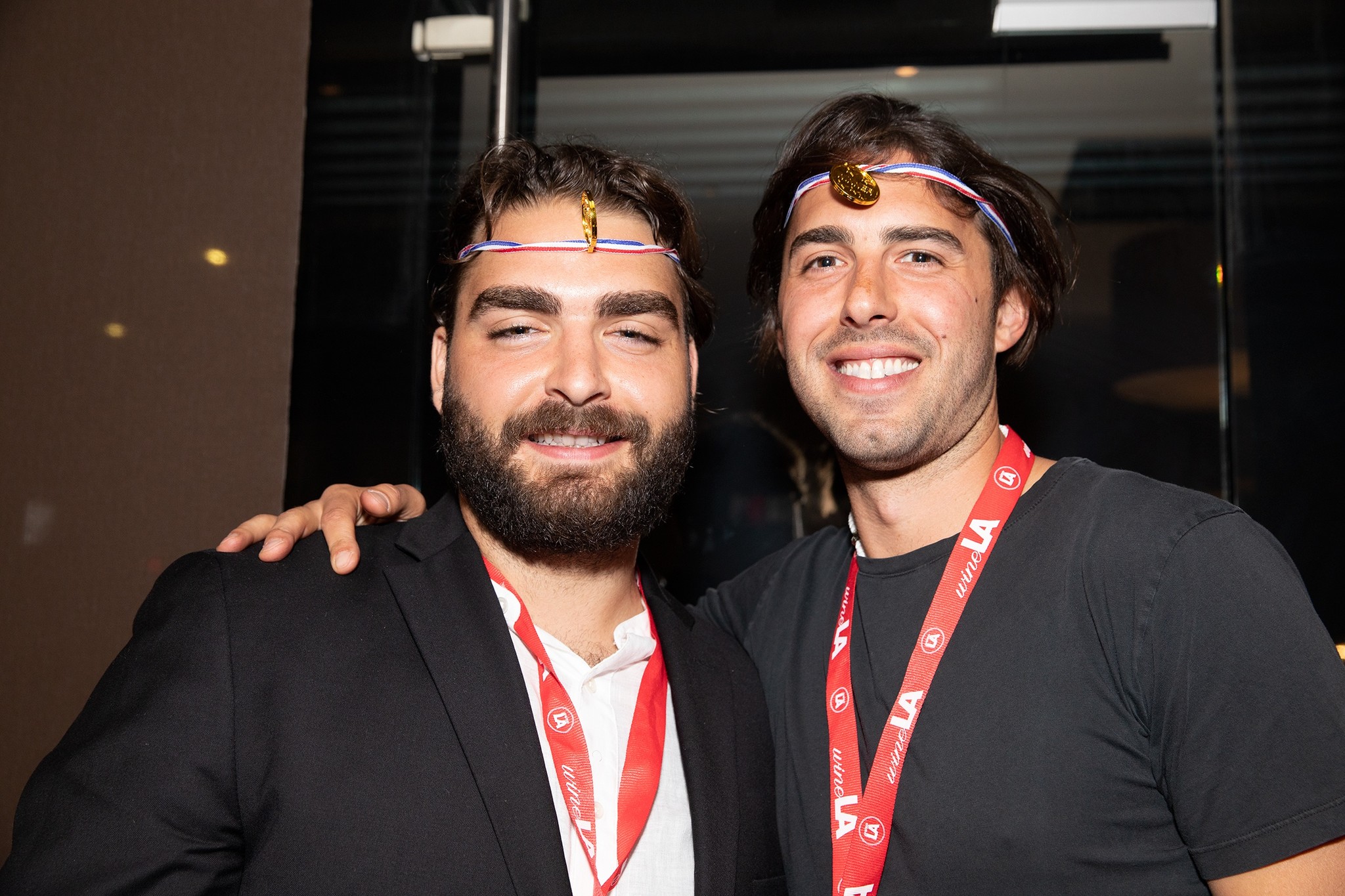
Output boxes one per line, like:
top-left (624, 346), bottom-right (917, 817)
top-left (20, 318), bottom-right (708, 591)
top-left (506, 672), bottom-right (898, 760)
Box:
top-left (5, 136), bottom-right (793, 894)
top-left (213, 86), bottom-right (1345, 896)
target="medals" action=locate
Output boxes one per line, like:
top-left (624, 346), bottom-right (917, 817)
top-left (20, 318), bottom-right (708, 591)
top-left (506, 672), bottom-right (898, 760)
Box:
top-left (828, 162), bottom-right (880, 206)
top-left (579, 191), bottom-right (602, 252)
